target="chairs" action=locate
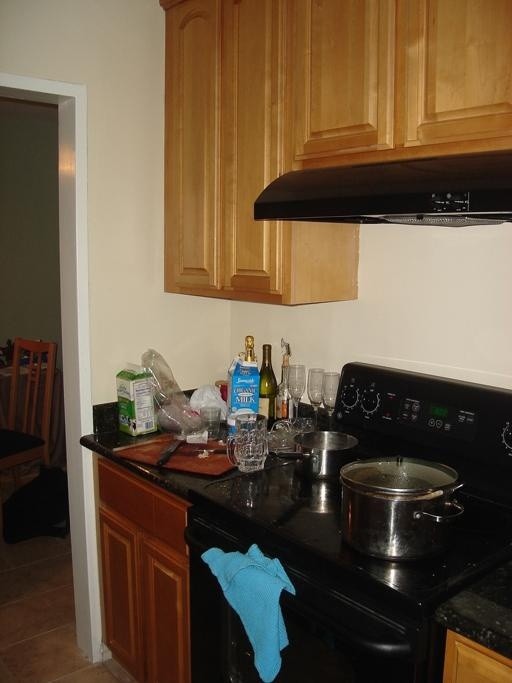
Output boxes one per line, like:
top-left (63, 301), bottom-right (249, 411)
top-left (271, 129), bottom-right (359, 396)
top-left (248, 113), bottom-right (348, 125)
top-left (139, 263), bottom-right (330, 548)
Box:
top-left (1, 337), bottom-right (57, 472)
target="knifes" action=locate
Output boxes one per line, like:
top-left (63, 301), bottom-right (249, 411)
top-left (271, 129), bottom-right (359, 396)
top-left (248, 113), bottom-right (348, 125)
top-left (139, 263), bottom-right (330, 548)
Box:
top-left (156, 441), bottom-right (186, 469)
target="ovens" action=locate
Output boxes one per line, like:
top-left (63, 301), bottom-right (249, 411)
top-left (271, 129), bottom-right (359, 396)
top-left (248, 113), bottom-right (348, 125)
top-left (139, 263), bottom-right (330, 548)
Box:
top-left (185, 505), bottom-right (429, 681)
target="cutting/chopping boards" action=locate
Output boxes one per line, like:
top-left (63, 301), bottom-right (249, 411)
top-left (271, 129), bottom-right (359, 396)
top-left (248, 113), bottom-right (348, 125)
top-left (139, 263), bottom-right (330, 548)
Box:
top-left (112, 434), bottom-right (238, 480)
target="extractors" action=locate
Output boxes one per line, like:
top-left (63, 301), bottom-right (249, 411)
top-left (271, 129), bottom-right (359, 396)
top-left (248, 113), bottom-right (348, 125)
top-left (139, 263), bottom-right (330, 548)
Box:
top-left (253, 146), bottom-right (512, 229)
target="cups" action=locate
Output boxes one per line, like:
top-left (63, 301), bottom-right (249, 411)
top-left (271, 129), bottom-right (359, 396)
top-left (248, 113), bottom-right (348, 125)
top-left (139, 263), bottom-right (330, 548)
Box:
top-left (197, 406), bottom-right (221, 442)
top-left (225, 413), bottom-right (269, 474)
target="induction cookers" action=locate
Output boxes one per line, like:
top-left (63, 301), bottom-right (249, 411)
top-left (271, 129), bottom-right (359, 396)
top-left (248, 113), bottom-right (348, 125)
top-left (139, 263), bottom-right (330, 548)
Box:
top-left (190, 461), bottom-right (512, 615)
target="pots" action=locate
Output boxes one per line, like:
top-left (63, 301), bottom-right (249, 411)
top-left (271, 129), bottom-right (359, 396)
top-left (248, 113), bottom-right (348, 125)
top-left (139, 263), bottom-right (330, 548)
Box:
top-left (277, 430), bottom-right (359, 477)
top-left (340, 455), bottom-right (466, 564)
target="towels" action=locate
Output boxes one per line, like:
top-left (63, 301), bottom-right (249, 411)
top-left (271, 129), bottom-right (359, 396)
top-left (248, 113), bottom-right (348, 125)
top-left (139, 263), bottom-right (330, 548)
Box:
top-left (201, 543), bottom-right (298, 683)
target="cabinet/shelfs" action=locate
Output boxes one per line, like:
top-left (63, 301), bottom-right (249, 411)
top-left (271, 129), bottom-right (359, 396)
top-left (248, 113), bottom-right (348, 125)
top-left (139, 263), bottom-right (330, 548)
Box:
top-left (158, 1), bottom-right (360, 305)
top-left (439, 628), bottom-right (512, 683)
top-left (287, 0), bottom-right (512, 167)
top-left (92, 449), bottom-right (195, 681)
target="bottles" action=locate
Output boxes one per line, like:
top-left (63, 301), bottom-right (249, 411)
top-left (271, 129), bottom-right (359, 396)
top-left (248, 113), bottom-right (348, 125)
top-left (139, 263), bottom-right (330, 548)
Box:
top-left (257, 344), bottom-right (290, 422)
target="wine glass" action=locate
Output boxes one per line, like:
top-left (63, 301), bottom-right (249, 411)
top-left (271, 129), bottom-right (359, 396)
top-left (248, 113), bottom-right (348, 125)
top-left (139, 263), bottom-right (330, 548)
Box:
top-left (286, 364), bottom-right (341, 433)
